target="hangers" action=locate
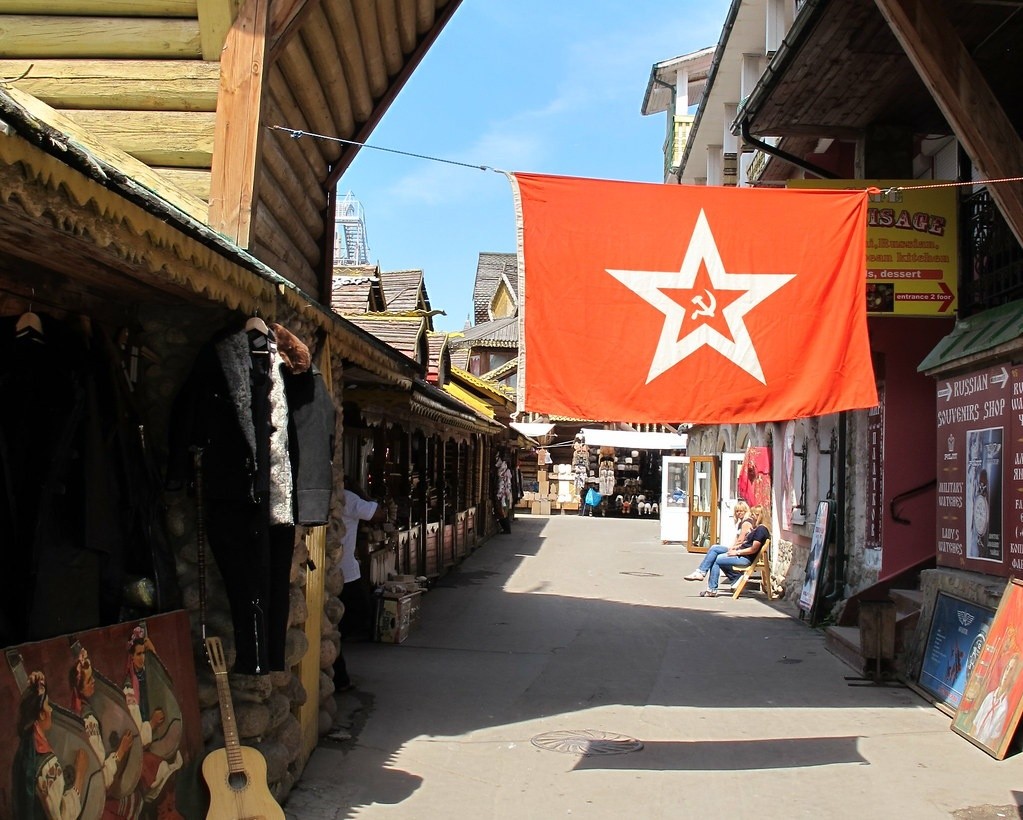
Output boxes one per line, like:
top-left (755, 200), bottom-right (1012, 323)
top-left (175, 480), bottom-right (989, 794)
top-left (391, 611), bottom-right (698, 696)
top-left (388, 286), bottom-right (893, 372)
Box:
top-left (244, 296), bottom-right (271, 354)
top-left (16, 285), bottom-right (42, 334)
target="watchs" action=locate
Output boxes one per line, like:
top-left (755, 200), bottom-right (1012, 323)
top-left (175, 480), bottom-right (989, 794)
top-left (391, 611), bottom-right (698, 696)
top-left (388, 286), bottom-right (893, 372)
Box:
top-left (972, 469), bottom-right (990, 558)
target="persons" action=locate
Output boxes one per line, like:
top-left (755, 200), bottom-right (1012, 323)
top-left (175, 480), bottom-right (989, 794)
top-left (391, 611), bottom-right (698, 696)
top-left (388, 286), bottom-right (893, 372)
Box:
top-left (333, 475), bottom-right (383, 691)
top-left (969, 654), bottom-right (1020, 747)
top-left (684, 501), bottom-right (771, 597)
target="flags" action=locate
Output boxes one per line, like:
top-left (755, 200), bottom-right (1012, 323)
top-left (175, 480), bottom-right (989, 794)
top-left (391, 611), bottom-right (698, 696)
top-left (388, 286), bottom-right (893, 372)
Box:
top-left (508, 171), bottom-right (879, 423)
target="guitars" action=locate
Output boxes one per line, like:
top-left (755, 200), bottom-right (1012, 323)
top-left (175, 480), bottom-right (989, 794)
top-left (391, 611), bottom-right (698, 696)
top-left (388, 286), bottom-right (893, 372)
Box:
top-left (197, 634), bottom-right (285, 820)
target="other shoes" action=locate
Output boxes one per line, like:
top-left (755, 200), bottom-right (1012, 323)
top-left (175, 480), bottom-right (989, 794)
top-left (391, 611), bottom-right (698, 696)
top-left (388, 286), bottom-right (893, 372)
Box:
top-left (684, 574), bottom-right (704, 581)
top-left (700, 591), bottom-right (716, 596)
top-left (333, 681), bottom-right (352, 691)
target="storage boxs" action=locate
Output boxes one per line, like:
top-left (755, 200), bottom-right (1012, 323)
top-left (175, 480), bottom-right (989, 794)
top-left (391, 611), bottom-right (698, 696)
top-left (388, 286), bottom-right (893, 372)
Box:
top-left (515, 470), bottom-right (578, 515)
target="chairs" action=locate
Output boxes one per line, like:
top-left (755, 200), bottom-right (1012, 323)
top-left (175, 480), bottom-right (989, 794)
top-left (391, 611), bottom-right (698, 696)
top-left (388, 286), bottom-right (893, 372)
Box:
top-left (730, 539), bottom-right (772, 600)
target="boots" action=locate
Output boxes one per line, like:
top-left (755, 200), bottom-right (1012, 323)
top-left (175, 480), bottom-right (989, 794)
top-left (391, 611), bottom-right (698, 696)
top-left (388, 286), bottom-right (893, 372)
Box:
top-left (499, 517), bottom-right (512, 534)
